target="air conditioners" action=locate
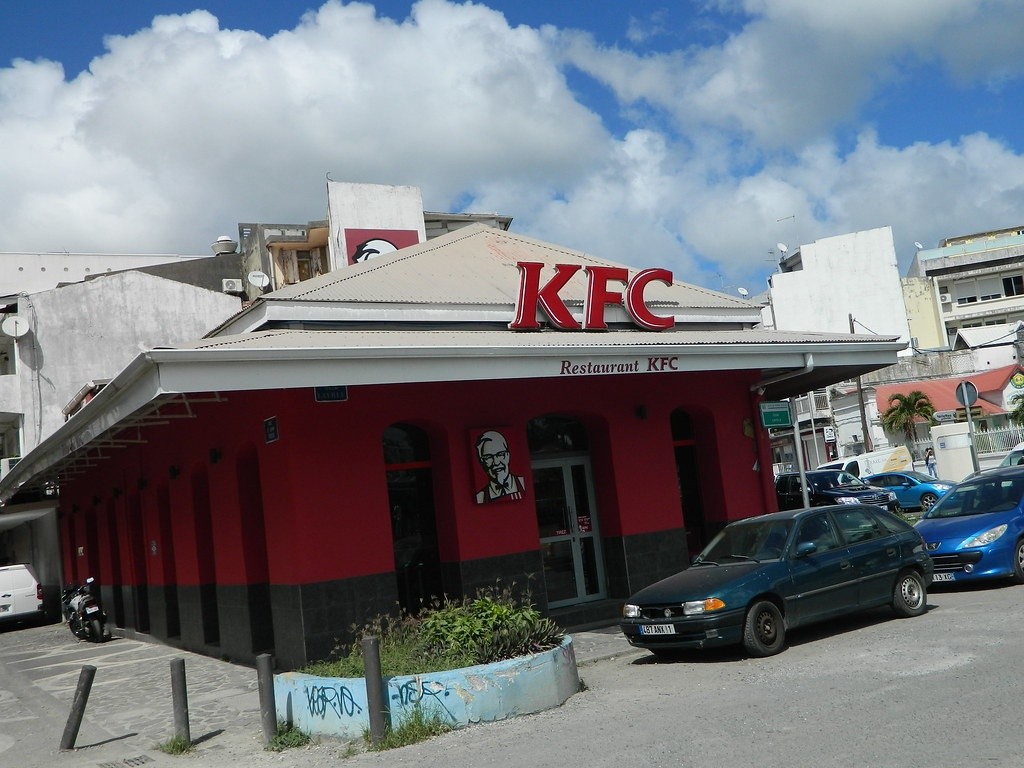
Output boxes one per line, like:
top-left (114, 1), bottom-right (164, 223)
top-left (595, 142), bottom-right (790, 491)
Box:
top-left (222, 278), bottom-right (244, 294)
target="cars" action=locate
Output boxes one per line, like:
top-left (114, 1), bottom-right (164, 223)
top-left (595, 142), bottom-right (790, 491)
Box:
top-left (913, 464), bottom-right (1024, 585)
top-left (861, 471), bottom-right (957, 512)
top-left (620, 502), bottom-right (934, 658)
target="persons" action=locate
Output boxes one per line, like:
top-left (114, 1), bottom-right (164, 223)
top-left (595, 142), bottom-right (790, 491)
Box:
top-left (925, 448), bottom-right (938, 479)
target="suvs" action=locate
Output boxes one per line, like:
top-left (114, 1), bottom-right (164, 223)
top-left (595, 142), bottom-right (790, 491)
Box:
top-left (775, 470), bottom-right (898, 512)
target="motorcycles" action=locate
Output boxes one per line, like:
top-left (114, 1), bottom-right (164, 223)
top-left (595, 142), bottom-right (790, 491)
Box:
top-left (60, 578), bottom-right (107, 644)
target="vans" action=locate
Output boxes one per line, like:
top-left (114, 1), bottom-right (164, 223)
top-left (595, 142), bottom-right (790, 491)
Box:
top-left (817, 446), bottom-right (914, 478)
top-left (0, 563), bottom-right (46, 623)
